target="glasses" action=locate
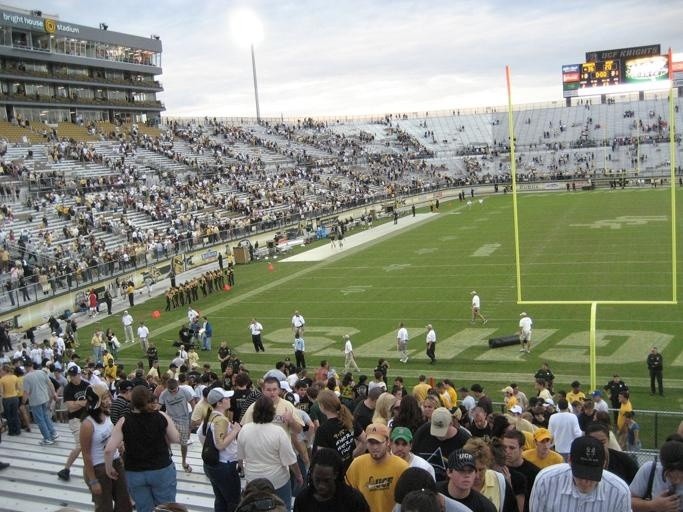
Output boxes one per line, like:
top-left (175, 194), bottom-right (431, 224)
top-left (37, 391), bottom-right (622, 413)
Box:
top-left (236, 498), bottom-right (275, 511)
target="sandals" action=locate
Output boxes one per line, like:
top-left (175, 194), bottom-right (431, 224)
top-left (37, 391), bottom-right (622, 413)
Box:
top-left (182, 463), bottom-right (192, 473)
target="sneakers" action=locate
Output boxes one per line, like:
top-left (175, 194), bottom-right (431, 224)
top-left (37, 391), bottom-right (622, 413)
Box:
top-left (52, 432), bottom-right (58, 439)
top-left (39, 438), bottom-right (55, 445)
top-left (0, 462), bottom-right (10, 471)
top-left (57, 469), bottom-right (69, 480)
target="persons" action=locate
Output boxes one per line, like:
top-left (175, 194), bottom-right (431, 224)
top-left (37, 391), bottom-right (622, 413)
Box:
top-left (1, 95), bottom-right (683, 512)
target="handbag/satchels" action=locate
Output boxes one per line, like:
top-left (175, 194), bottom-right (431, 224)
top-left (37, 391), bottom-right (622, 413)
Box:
top-left (202, 430), bottom-right (219, 468)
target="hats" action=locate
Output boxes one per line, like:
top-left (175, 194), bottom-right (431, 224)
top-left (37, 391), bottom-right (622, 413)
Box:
top-left (364, 385), bottom-right (605, 443)
top-left (470, 291), bottom-right (477, 295)
top-left (570, 436), bottom-right (604, 481)
top-left (520, 312), bottom-right (527, 316)
top-left (208, 387), bottom-right (234, 405)
top-left (448, 449), bottom-right (477, 473)
top-left (85, 382), bottom-right (106, 411)
top-left (66, 366), bottom-right (81, 375)
top-left (169, 363), bottom-right (178, 369)
top-left (425, 324), bottom-right (432, 328)
top-left (343, 335), bottom-right (350, 338)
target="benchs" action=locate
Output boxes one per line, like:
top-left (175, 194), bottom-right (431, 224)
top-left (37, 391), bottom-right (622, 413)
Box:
top-left (0, 416), bottom-right (289, 512)
